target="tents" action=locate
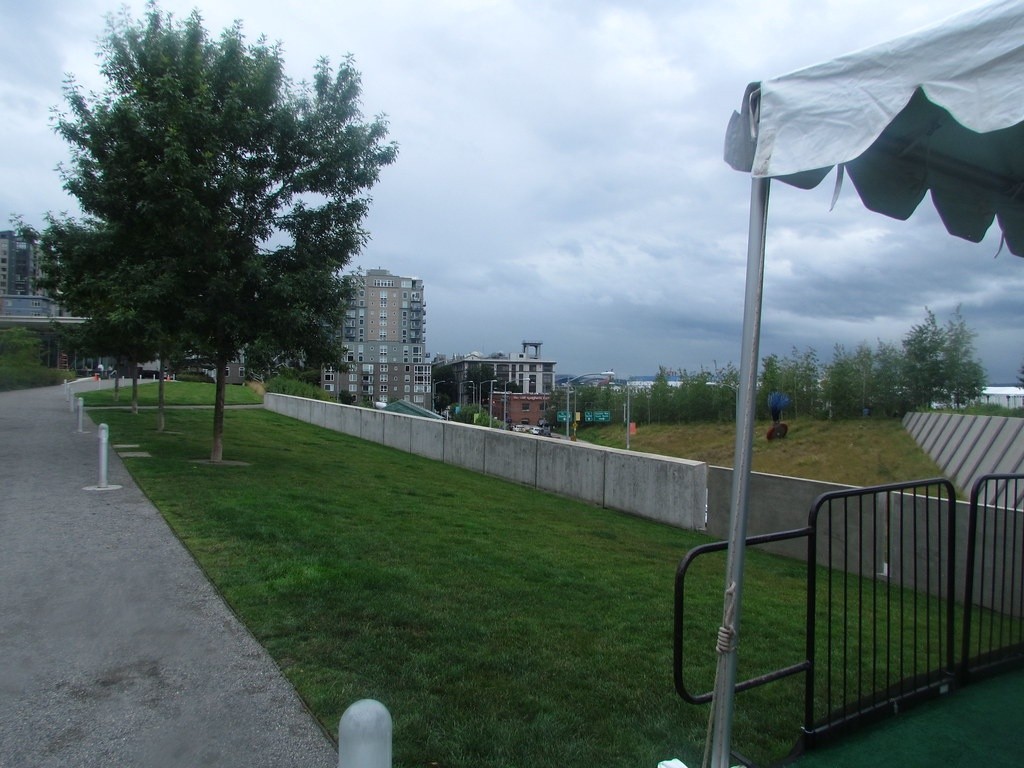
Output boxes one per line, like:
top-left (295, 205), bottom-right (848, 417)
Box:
top-left (705, 0), bottom-right (1023, 768)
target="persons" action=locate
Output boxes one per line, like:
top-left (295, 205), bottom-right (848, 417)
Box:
top-left (97, 363), bottom-right (104, 380)
top-left (107, 366), bottom-right (113, 377)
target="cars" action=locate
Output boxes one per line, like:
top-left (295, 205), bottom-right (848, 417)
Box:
top-left (513, 424), bottom-right (524, 432)
top-left (524, 425), bottom-right (552, 437)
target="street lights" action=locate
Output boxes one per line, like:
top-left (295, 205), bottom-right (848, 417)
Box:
top-left (609, 382), bottom-right (630, 450)
top-left (561, 383), bottom-right (576, 441)
top-left (479, 380), bottom-right (497, 428)
top-left (459, 381), bottom-right (474, 411)
top-left (504, 378), bottom-right (531, 429)
top-left (705, 381), bottom-right (738, 435)
top-left (566, 372), bottom-right (615, 440)
top-left (434, 381), bottom-right (446, 395)
